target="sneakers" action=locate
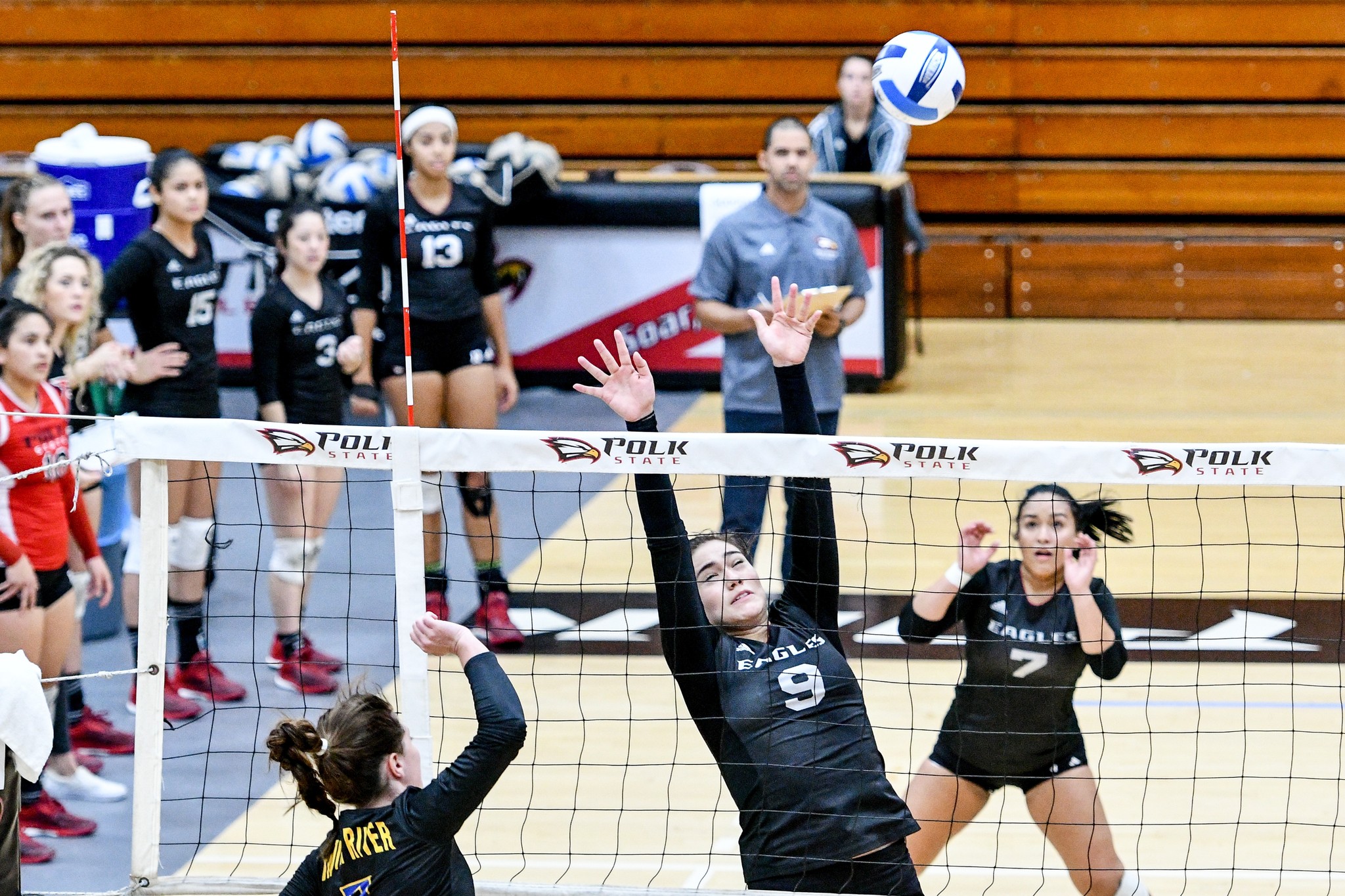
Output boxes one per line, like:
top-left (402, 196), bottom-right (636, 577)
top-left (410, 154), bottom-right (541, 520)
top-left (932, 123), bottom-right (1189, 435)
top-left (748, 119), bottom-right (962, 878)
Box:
top-left (73, 745), bottom-right (104, 771)
top-left (469, 591), bottom-right (524, 645)
top-left (127, 670), bottom-right (201, 721)
top-left (174, 649), bottom-right (247, 701)
top-left (273, 642), bottom-right (339, 696)
top-left (20, 791), bottom-right (97, 838)
top-left (69, 707), bottom-right (134, 754)
top-left (423, 591), bottom-right (449, 621)
top-left (266, 629), bottom-right (344, 672)
top-left (18, 824), bottom-right (54, 864)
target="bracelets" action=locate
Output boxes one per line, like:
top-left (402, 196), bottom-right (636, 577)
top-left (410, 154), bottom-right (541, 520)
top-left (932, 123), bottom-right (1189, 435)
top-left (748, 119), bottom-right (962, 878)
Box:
top-left (836, 314), bottom-right (846, 337)
top-left (945, 563), bottom-right (971, 590)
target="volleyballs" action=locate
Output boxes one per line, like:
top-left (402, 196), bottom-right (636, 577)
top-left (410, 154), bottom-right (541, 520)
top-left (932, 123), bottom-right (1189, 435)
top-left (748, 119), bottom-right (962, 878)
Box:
top-left (220, 118), bottom-right (398, 205)
top-left (871, 30), bottom-right (966, 125)
top-left (442, 156), bottom-right (489, 173)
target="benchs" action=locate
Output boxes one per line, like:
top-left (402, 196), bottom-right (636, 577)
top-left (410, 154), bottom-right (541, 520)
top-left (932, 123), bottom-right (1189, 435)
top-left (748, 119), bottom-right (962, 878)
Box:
top-left (0, 0), bottom-right (1345, 320)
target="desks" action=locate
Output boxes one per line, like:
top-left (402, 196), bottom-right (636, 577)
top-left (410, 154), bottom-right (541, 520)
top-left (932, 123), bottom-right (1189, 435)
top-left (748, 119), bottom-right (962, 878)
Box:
top-left (209, 169), bottom-right (906, 392)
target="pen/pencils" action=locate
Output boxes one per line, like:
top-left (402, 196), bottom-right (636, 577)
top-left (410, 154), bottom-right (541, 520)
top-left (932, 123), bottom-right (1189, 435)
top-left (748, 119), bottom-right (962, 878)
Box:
top-left (757, 292), bottom-right (770, 308)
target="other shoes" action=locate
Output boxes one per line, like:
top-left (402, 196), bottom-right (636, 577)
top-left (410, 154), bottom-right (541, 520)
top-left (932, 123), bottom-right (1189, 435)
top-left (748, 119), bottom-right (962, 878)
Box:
top-left (42, 764), bottom-right (126, 802)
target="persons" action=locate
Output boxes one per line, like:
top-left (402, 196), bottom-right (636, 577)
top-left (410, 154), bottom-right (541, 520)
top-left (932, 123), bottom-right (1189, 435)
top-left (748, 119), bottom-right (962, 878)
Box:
top-left (897, 483), bottom-right (1151, 895)
top-left (572, 276), bottom-right (926, 896)
top-left (97, 147), bottom-right (247, 723)
top-left (686, 113), bottom-right (871, 587)
top-left (0, 173), bottom-right (135, 867)
top-left (268, 612), bottom-right (528, 896)
top-left (804, 54), bottom-right (929, 395)
top-left (251, 202), bottom-right (364, 694)
top-left (345, 96), bottom-right (526, 651)
